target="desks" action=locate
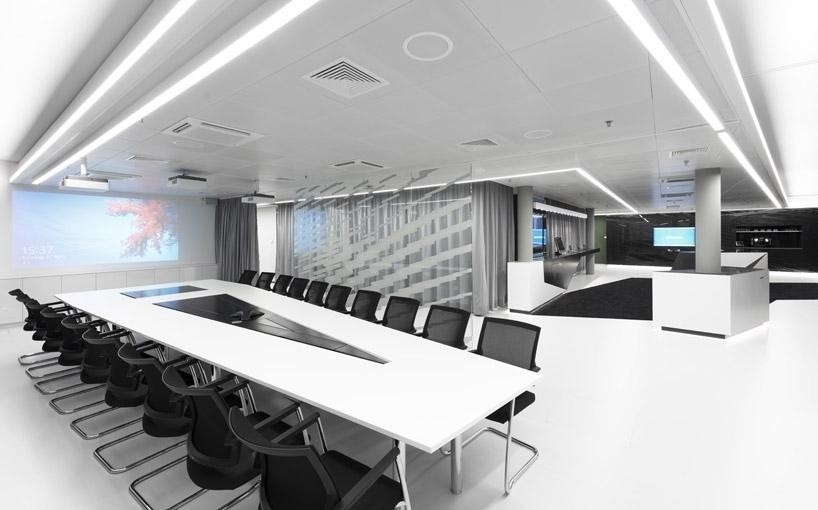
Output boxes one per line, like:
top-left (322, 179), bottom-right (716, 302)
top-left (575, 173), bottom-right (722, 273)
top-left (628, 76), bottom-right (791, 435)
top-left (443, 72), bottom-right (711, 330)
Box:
top-left (544, 248), bottom-right (601, 263)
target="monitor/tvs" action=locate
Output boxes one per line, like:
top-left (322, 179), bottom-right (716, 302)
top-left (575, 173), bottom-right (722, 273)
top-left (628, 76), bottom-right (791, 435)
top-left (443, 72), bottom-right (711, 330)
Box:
top-left (653, 226), bottom-right (696, 247)
top-left (554, 237), bottom-right (564, 255)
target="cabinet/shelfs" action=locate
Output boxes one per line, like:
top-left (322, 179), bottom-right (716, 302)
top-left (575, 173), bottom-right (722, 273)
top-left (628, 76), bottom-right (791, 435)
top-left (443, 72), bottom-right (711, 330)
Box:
top-left (736, 230), bottom-right (804, 250)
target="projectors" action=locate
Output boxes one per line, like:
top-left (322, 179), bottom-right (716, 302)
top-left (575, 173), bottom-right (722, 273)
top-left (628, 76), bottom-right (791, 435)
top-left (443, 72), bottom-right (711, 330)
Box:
top-left (242, 194), bottom-right (274, 203)
top-left (168, 176), bottom-right (207, 186)
top-left (59, 176), bottom-right (108, 193)
